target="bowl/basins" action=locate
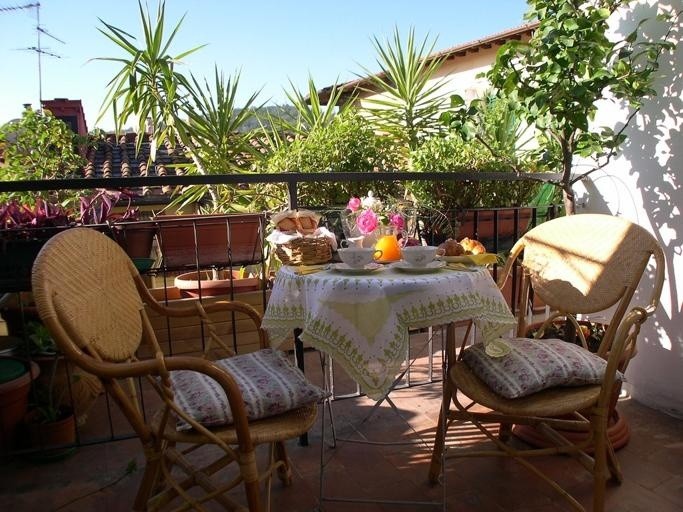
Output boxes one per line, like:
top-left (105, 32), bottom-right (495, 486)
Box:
top-left (0, 335), bottom-right (41, 422)
top-left (173, 270), bottom-right (261, 299)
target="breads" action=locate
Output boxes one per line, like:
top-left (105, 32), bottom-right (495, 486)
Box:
top-left (436, 237), bottom-right (485, 256)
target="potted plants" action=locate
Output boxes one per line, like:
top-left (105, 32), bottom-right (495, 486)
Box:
top-left (477, 0), bottom-right (682, 457)
top-left (406, 134), bottom-right (545, 247)
top-left (0, 0), bottom-right (262, 303)
top-left (0, 323), bottom-right (77, 461)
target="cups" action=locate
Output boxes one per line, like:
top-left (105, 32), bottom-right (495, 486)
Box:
top-left (336, 247), bottom-right (382, 269)
top-left (400, 246), bottom-right (446, 266)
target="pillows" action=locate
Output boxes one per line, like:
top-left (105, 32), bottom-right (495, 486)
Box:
top-left (460, 333), bottom-right (627, 396)
top-left (151, 345), bottom-right (329, 433)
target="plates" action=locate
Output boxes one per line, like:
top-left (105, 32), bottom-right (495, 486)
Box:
top-left (391, 262), bottom-right (448, 274)
top-left (329, 262), bottom-right (382, 273)
top-left (436, 253), bottom-right (499, 265)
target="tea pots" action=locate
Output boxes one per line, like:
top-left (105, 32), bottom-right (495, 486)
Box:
top-left (372, 227), bottom-right (408, 263)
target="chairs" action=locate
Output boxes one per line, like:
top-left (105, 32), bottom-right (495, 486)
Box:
top-left (31, 225), bottom-right (324, 510)
top-left (426, 212), bottom-right (666, 512)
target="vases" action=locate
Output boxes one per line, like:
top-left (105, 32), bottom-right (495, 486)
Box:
top-left (342, 215), bottom-right (383, 246)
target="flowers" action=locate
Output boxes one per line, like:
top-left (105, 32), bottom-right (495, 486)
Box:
top-left (339, 194), bottom-right (407, 236)
